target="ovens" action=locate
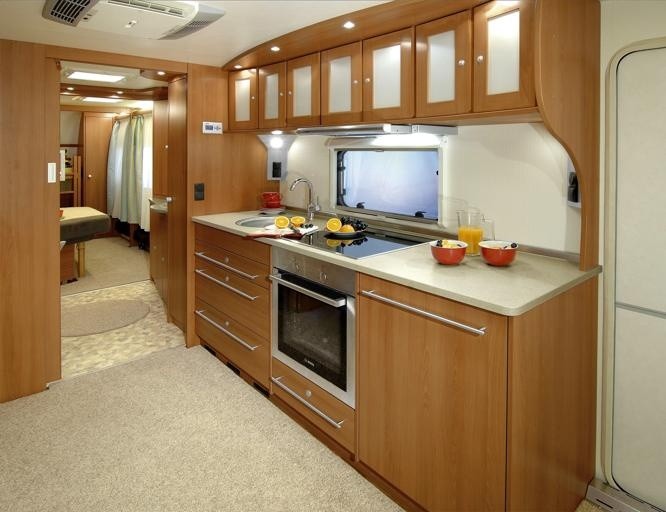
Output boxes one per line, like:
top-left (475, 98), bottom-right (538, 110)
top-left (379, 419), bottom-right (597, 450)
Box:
top-left (269, 246), bottom-right (356, 409)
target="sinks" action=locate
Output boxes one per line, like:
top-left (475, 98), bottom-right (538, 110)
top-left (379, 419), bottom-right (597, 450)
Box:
top-left (234, 214), bottom-right (293, 229)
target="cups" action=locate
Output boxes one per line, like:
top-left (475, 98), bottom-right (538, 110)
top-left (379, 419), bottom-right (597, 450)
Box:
top-left (262, 191), bottom-right (283, 208)
top-left (456, 208), bottom-right (495, 257)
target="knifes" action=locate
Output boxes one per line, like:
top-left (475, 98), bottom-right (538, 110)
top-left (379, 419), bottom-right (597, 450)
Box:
top-left (288, 224), bottom-right (300, 233)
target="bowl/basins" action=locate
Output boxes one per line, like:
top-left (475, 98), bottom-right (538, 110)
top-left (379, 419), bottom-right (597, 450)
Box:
top-left (428, 239), bottom-right (468, 265)
top-left (478, 240), bottom-right (519, 266)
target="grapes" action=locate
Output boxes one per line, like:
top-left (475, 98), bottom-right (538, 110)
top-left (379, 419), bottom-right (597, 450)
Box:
top-left (340, 215), bottom-right (368, 231)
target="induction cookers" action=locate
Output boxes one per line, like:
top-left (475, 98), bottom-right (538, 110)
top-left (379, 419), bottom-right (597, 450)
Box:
top-left (280, 226), bottom-right (435, 259)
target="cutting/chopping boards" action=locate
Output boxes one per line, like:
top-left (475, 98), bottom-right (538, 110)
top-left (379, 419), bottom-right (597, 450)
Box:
top-left (265, 223), bottom-right (318, 234)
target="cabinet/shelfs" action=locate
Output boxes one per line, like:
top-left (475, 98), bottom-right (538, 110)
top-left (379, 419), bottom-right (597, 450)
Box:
top-left (192, 224), bottom-right (271, 402)
top-left (414, 0), bottom-right (537, 126)
top-left (79, 113), bottom-right (112, 210)
top-left (258, 52), bottom-right (321, 129)
top-left (271, 356), bottom-right (356, 468)
top-left (228, 68), bottom-right (257, 130)
top-left (357, 276), bottom-right (597, 512)
top-left (321, 26), bottom-right (415, 124)
top-left (149, 198), bottom-right (169, 314)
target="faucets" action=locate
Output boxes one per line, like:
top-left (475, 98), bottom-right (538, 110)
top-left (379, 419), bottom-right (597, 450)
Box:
top-left (289, 178), bottom-right (321, 219)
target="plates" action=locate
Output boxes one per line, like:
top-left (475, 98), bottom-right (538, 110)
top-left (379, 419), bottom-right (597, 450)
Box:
top-left (259, 210), bottom-right (284, 215)
top-left (324, 225), bottom-right (368, 236)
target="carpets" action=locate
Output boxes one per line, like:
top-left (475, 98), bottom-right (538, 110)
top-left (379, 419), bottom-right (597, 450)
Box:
top-left (61, 235), bottom-right (150, 298)
top-left (0, 343), bottom-right (609, 511)
top-left (61, 300), bottom-right (149, 337)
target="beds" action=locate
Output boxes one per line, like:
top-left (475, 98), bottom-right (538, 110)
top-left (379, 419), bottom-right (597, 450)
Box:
top-left (60, 207), bottom-right (114, 279)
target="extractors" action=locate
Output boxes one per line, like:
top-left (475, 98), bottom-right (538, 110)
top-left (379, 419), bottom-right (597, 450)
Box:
top-left (296, 122), bottom-right (458, 137)
